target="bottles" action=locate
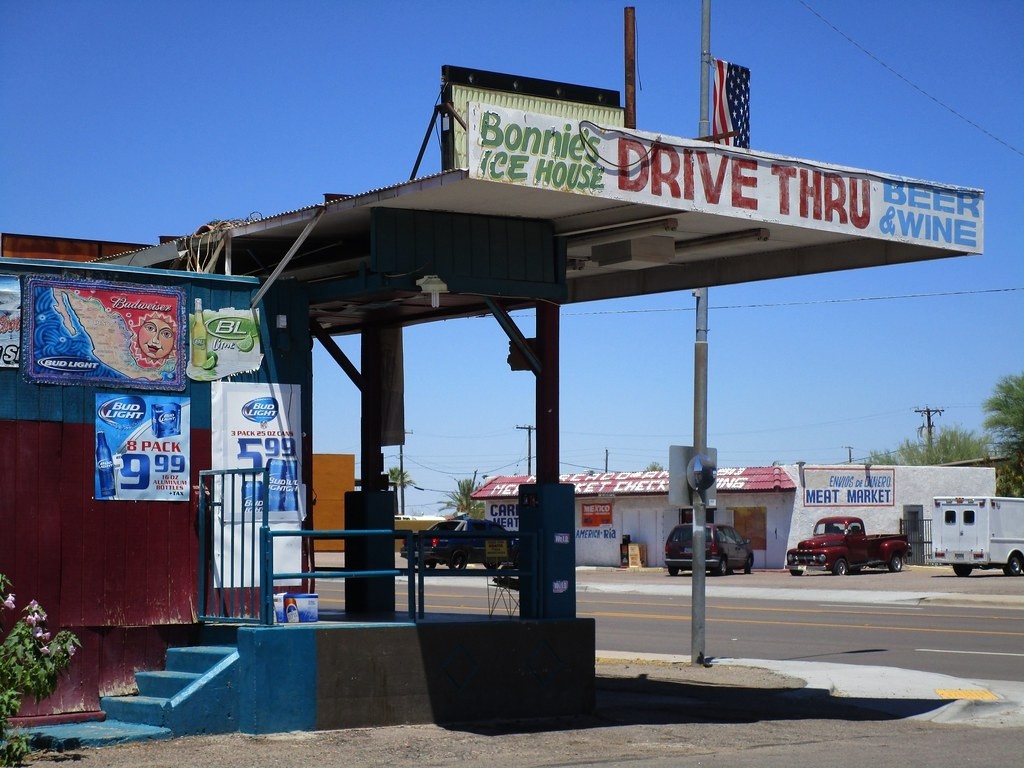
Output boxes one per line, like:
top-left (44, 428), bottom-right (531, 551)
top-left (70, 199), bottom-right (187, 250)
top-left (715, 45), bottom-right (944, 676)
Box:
top-left (96, 429), bottom-right (115, 496)
top-left (191, 296), bottom-right (208, 369)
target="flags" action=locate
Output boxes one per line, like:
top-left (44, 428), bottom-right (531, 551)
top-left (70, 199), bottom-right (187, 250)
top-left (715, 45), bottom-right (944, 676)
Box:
top-left (713, 60), bottom-right (750, 147)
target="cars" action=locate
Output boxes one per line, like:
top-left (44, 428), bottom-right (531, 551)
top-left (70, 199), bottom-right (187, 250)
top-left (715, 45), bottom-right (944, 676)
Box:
top-left (664, 523), bottom-right (754, 576)
top-left (401, 518), bottom-right (522, 571)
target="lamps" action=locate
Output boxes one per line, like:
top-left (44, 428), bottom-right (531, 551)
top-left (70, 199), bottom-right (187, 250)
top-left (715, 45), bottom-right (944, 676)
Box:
top-left (559, 217), bottom-right (678, 249)
top-left (675, 228), bottom-right (771, 254)
top-left (415, 275), bottom-right (451, 307)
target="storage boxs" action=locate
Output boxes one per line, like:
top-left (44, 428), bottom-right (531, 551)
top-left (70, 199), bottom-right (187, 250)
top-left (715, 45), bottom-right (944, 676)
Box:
top-left (274, 592), bottom-right (318, 623)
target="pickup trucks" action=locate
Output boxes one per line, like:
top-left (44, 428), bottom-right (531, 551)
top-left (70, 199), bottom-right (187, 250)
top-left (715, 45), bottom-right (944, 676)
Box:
top-left (785, 517), bottom-right (913, 577)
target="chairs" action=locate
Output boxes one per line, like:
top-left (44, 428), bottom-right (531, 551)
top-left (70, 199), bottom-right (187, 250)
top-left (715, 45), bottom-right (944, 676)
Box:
top-left (826, 526), bottom-right (840, 533)
top-left (850, 526), bottom-right (860, 534)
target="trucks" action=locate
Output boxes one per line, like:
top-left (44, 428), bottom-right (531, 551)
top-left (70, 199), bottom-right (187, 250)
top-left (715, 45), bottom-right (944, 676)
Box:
top-left (926, 496), bottom-right (1024, 577)
